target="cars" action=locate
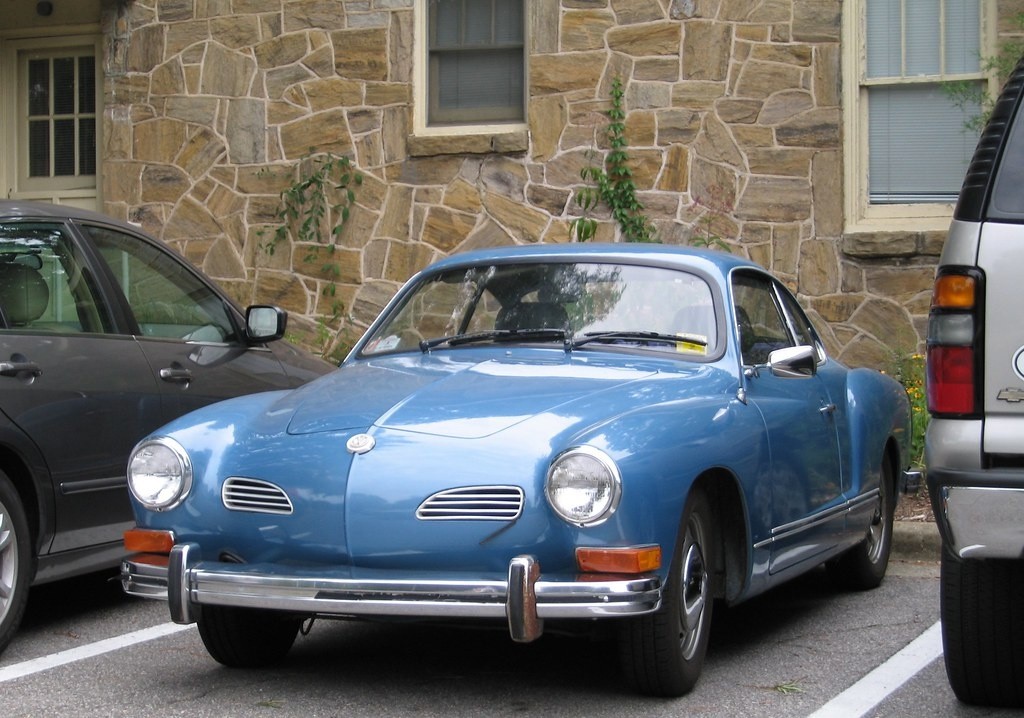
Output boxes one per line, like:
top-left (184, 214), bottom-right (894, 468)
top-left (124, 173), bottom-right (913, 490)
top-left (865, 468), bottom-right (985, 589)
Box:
top-left (0, 200), bottom-right (340, 653)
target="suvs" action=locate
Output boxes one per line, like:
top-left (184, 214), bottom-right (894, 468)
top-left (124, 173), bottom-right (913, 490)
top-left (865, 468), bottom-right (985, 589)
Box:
top-left (918, 67), bottom-right (1024, 709)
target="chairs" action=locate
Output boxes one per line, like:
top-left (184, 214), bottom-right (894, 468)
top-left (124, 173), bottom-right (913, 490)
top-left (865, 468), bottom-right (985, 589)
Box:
top-left (0, 263), bottom-right (80, 333)
top-left (670, 305), bottom-right (757, 361)
top-left (495, 302), bottom-right (573, 342)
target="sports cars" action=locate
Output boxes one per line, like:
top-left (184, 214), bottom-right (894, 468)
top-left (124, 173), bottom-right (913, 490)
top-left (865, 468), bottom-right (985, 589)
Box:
top-left (118, 244), bottom-right (915, 702)
